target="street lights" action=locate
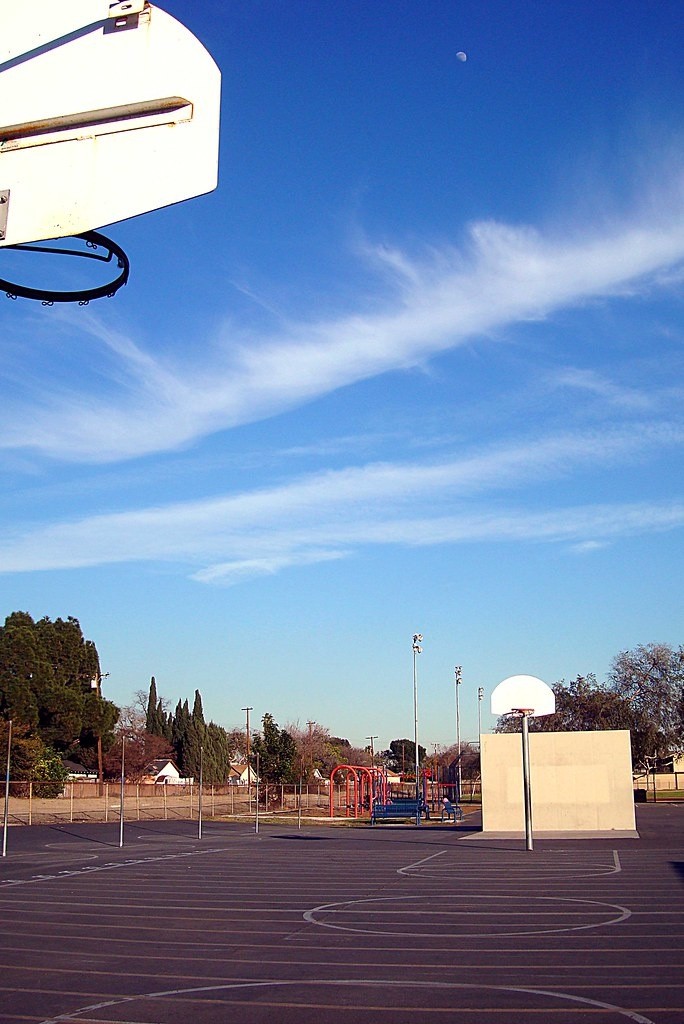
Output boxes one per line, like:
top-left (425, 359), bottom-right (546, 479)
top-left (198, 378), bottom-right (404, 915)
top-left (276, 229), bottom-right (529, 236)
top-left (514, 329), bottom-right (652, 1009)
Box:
top-left (410, 631), bottom-right (424, 787)
top-left (453, 665), bottom-right (464, 799)
top-left (477, 685), bottom-right (484, 741)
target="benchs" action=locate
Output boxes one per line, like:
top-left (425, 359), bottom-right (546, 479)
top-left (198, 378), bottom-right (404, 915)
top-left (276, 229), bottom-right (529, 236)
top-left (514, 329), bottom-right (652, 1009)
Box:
top-left (372, 805), bottom-right (423, 825)
top-left (442, 797), bottom-right (463, 824)
